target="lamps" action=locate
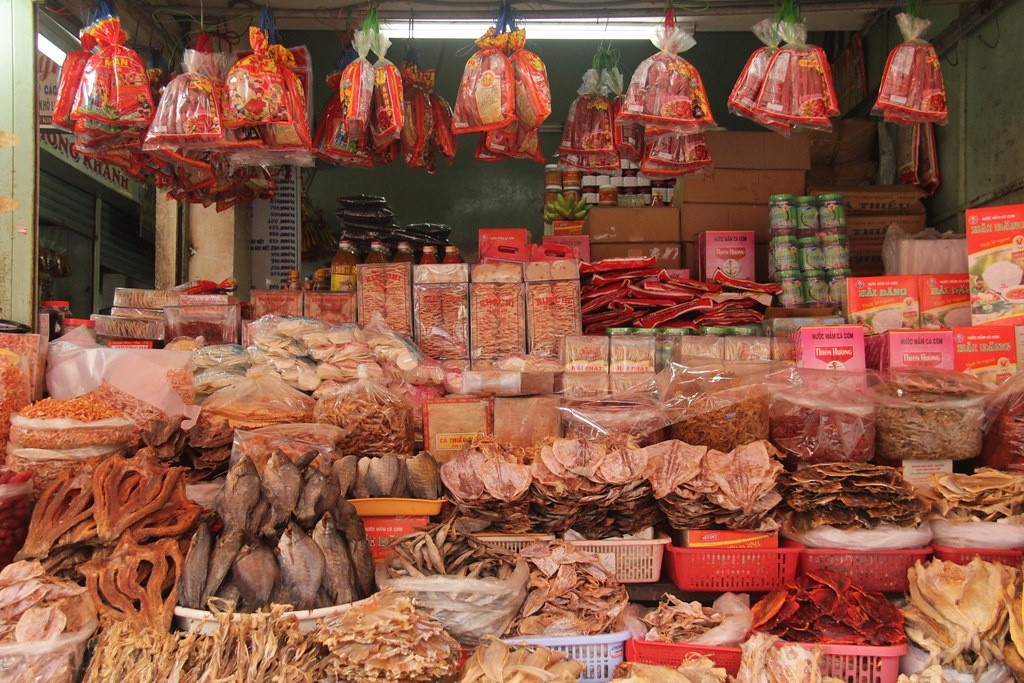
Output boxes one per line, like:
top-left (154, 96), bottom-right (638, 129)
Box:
top-left (354, 15), bottom-right (698, 50)
top-left (34, 16), bottom-right (74, 69)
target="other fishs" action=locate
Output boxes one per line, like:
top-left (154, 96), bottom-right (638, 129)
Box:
top-left (176, 448), bottom-right (378, 615)
top-left (332, 450), bottom-right (441, 499)
top-left (746, 569), bottom-right (906, 660)
top-left (383, 501), bottom-right (527, 645)
top-left (898, 553), bottom-right (1024, 683)
top-left (0, 558), bottom-right (96, 683)
top-left (439, 433), bottom-right (785, 538)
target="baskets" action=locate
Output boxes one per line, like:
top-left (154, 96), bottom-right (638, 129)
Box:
top-left (475, 533), bottom-right (555, 553)
top-left (503, 632), bottom-right (632, 683)
top-left (625, 637), bottom-right (742, 683)
top-left (799, 545), bottom-right (933, 591)
top-left (772, 639), bottom-right (907, 683)
top-left (934, 543), bottom-right (1024, 567)
top-left (564, 531), bottom-right (671, 584)
top-left (664, 542), bottom-right (804, 591)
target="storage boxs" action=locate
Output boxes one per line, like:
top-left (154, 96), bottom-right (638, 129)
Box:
top-left (552, 219), bottom-right (584, 235)
top-left (810, 185), bottom-right (930, 278)
top-left (865, 334), bottom-right (871, 364)
top-left (663, 542), bottom-right (805, 596)
top-left (680, 528), bottom-right (784, 581)
top-left (841, 271), bottom-right (921, 332)
top-left (883, 330), bottom-right (955, 376)
top-left (949, 326), bottom-right (1015, 385)
top-left (542, 234), bottom-right (591, 263)
top-left (791, 323), bottom-right (868, 390)
top-left (504, 630), bottom-right (631, 683)
top-left (695, 233), bottom-right (704, 281)
top-left (362, 515), bottom-right (431, 563)
top-left (917, 273), bottom-right (970, 329)
top-left (678, 206), bottom-right (774, 235)
top-left (800, 544), bottom-right (935, 593)
top-left (624, 636), bottom-right (741, 680)
top-left (699, 127), bottom-right (823, 171)
top-left (563, 528), bottom-right (672, 583)
top-left (682, 169), bottom-right (808, 205)
top-left (931, 547), bottom-right (1024, 571)
top-left (963, 203), bottom-right (1024, 326)
top-left (706, 229), bottom-right (756, 283)
top-left (774, 636), bottom-right (909, 683)
top-left (682, 240), bottom-right (695, 270)
top-left (478, 533), bottom-right (559, 554)
top-left (871, 333), bottom-right (883, 373)
top-left (476, 227), bottom-right (527, 263)
top-left (589, 206), bottom-right (676, 244)
top-left (590, 242), bottom-right (682, 267)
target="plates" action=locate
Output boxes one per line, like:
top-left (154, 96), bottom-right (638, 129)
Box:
top-left (1003, 285), bottom-right (1024, 303)
top-left (872, 311), bottom-right (903, 332)
top-left (944, 308), bottom-right (971, 327)
top-left (982, 261), bottom-right (1022, 292)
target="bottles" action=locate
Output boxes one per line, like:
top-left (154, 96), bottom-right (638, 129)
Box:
top-left (698, 327), bottom-right (729, 335)
top-left (769, 193), bottom-right (852, 307)
top-left (63, 318), bottom-right (96, 341)
top-left (632, 328), bottom-right (659, 335)
top-left (660, 328), bottom-right (689, 335)
top-left (545, 160), bottom-right (675, 207)
top-left (729, 326), bottom-right (764, 336)
top-left (606, 328), bottom-right (631, 335)
top-left (289, 240), bottom-right (462, 292)
top-left (39, 300), bottom-right (71, 338)
